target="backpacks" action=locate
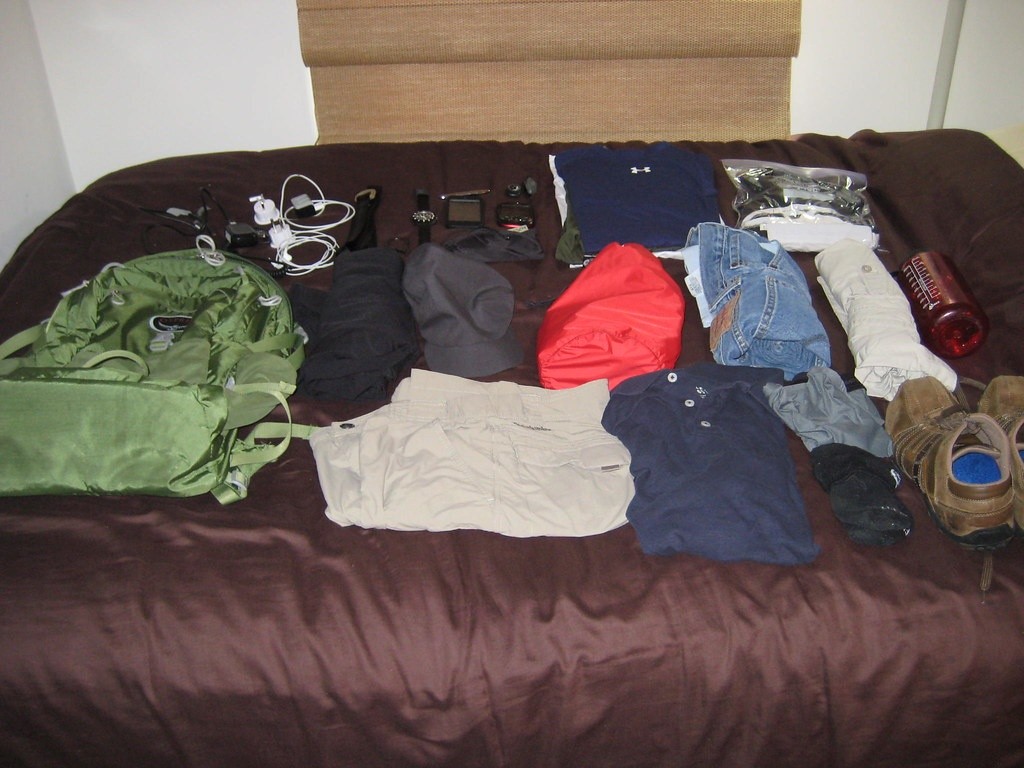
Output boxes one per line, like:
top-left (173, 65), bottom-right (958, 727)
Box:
top-left (1, 242), bottom-right (309, 507)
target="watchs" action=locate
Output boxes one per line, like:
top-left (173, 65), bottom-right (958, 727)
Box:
top-left (410, 188), bottom-right (437, 249)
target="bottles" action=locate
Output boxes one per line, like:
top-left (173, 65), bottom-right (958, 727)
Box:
top-left (890, 247), bottom-right (987, 359)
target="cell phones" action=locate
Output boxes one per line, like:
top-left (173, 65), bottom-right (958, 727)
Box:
top-left (445, 195), bottom-right (485, 229)
top-left (496, 202), bottom-right (535, 228)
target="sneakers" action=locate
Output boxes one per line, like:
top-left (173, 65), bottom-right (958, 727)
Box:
top-left (971, 373), bottom-right (1024, 533)
top-left (885, 373), bottom-right (1016, 550)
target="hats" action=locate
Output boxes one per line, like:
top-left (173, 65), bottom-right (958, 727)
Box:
top-left (406, 241), bottom-right (524, 382)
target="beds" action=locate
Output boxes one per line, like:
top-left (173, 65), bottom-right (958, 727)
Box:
top-left (0, 126), bottom-right (1021, 768)
